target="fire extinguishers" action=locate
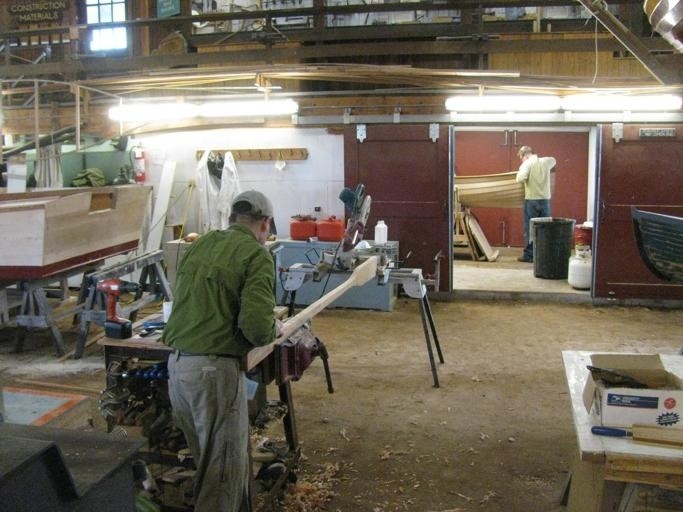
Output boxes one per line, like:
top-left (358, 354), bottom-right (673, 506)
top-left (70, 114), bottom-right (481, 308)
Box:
top-left (129, 142), bottom-right (146, 182)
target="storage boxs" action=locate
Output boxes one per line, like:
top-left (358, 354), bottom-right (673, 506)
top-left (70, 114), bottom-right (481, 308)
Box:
top-left (582, 354), bottom-right (683, 427)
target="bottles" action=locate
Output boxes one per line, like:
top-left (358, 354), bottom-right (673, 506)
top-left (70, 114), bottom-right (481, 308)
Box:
top-left (374, 218), bottom-right (388, 246)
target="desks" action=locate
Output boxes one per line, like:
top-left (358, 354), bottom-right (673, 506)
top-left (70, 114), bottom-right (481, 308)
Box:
top-left (562, 349), bottom-right (683, 512)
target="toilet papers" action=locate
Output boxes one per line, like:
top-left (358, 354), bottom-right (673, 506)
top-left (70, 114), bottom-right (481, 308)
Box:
top-left (5, 164), bottom-right (27, 193)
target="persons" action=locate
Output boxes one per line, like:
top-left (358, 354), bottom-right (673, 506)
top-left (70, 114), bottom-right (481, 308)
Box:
top-left (161, 190), bottom-right (285, 512)
top-left (516, 146), bottom-right (557, 263)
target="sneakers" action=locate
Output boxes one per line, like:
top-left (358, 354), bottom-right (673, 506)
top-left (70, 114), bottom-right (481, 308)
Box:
top-left (518, 254), bottom-right (533, 263)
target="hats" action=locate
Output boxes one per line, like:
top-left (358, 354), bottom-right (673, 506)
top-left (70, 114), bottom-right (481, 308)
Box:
top-left (231, 190), bottom-right (277, 235)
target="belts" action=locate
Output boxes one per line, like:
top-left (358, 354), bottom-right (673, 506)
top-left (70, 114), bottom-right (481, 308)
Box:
top-left (172, 349), bottom-right (234, 359)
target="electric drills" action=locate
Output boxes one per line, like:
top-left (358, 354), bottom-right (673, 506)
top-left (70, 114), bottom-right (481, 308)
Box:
top-left (96, 278), bottom-right (145, 340)
